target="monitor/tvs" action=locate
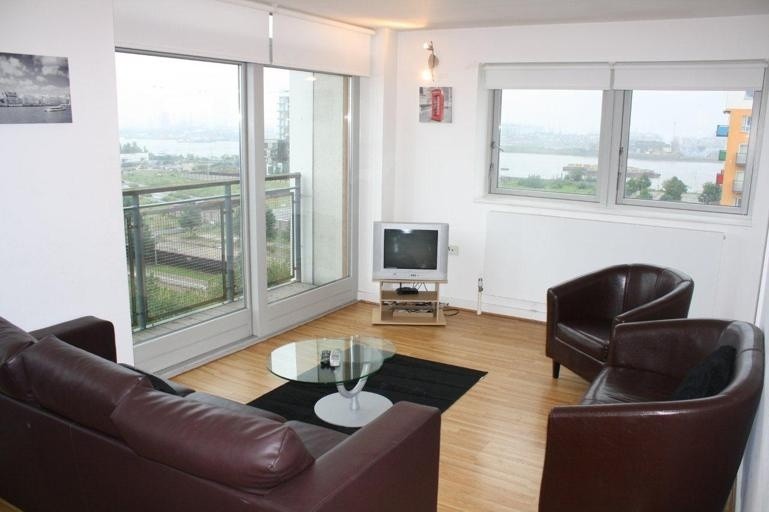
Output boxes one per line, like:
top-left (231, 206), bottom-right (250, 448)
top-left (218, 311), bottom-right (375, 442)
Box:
top-left (372, 221), bottom-right (449, 281)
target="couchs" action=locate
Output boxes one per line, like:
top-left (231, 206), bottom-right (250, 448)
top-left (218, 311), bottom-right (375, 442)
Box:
top-left (1, 315), bottom-right (440, 512)
top-left (545, 264), bottom-right (695, 384)
top-left (537, 318), bottom-right (765, 512)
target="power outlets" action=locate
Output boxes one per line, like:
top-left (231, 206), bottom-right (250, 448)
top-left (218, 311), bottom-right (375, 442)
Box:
top-left (449, 246), bottom-right (459, 256)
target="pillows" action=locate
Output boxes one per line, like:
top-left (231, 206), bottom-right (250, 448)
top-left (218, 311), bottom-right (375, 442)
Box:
top-left (119, 362), bottom-right (179, 397)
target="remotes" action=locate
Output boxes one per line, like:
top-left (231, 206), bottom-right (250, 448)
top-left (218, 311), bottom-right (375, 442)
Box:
top-left (330, 348), bottom-right (342, 368)
top-left (320, 350), bottom-right (330, 366)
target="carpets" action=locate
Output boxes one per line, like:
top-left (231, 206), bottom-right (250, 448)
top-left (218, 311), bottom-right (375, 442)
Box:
top-left (246, 345), bottom-right (489, 435)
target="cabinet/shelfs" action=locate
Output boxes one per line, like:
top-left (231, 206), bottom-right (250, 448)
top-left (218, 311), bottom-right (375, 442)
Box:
top-left (370, 280), bottom-right (448, 326)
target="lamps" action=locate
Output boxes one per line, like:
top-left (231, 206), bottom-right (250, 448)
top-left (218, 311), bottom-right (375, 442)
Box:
top-left (421, 40), bottom-right (438, 84)
